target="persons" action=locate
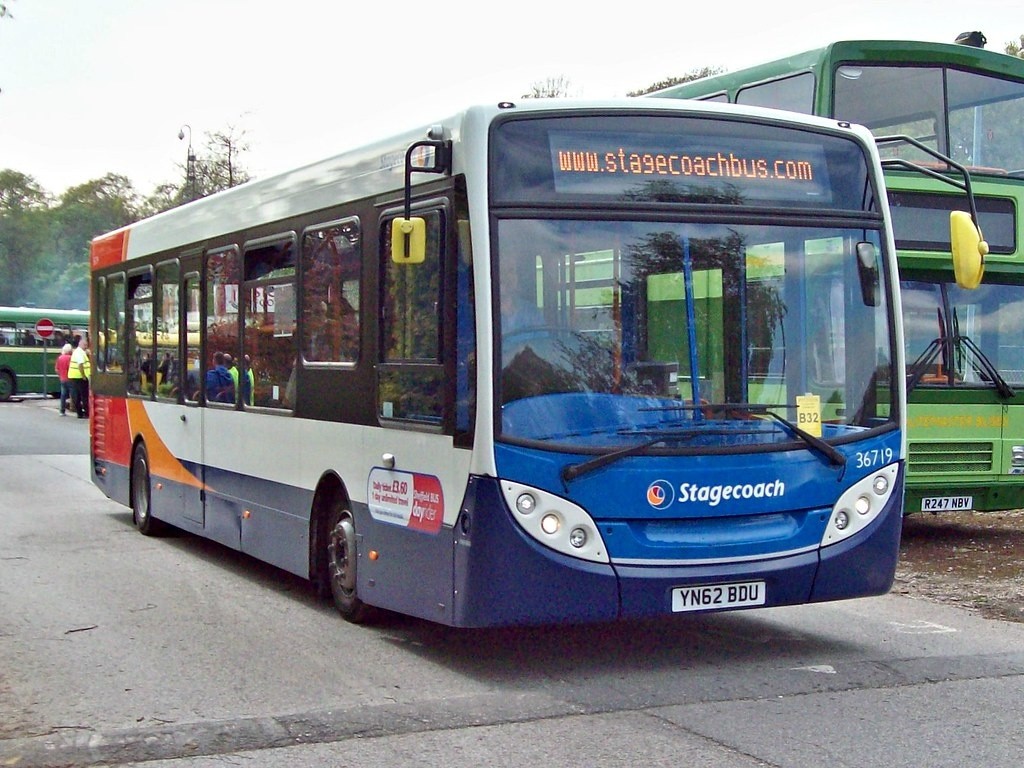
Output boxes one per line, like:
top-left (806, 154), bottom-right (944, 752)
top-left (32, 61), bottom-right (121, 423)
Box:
top-left (55, 340), bottom-right (90, 418)
top-left (140, 351), bottom-right (255, 406)
top-left (21, 330), bottom-right (35, 345)
top-left (53, 331), bottom-right (81, 347)
top-left (466, 239), bottom-right (549, 348)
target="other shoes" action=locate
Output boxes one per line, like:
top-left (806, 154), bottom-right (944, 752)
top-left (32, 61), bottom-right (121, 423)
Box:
top-left (77, 413), bottom-right (86, 418)
top-left (59, 412), bottom-right (66, 416)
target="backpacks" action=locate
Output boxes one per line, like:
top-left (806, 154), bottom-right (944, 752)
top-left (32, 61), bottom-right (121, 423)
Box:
top-left (212, 371), bottom-right (237, 404)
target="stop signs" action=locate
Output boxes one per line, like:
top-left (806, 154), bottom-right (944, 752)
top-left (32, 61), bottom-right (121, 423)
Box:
top-left (37, 320), bottom-right (55, 338)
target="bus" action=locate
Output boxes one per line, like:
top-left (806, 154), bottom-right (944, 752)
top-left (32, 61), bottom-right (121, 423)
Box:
top-left (90, 99), bottom-right (989, 629)
top-left (537, 39), bottom-right (1024, 513)
top-left (1, 306), bottom-right (129, 400)
